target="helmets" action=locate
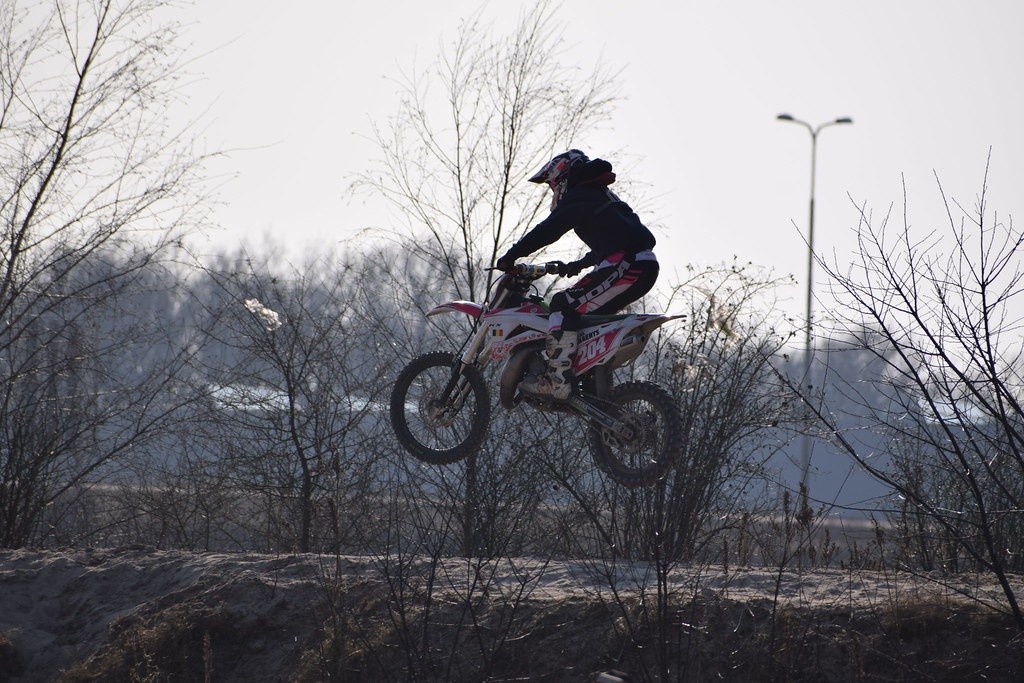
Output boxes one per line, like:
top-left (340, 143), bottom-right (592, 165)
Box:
top-left (528, 149), bottom-right (591, 211)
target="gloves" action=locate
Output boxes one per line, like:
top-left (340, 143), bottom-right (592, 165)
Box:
top-left (566, 261), bottom-right (584, 278)
top-left (496, 254), bottom-right (515, 274)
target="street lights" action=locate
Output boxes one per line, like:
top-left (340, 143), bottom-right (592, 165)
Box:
top-left (777, 111), bottom-right (854, 516)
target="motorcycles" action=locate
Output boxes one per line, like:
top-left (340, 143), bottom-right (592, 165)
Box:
top-left (390, 262), bottom-right (685, 489)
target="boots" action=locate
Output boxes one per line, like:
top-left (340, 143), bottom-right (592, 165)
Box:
top-left (517, 331), bottom-right (579, 404)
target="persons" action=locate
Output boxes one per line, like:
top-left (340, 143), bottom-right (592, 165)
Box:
top-left (497, 148), bottom-right (660, 404)
